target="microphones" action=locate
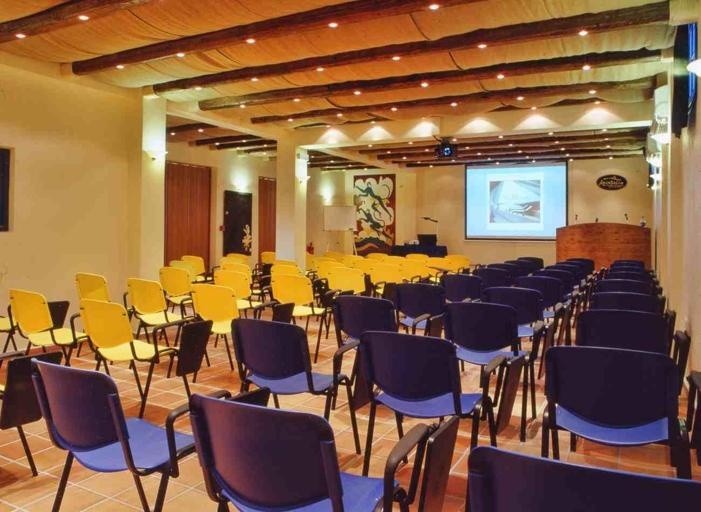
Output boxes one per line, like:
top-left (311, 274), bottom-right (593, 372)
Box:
top-left (575, 215), bottom-right (577, 220)
top-left (595, 218), bottom-right (598, 223)
top-left (624, 213), bottom-right (628, 220)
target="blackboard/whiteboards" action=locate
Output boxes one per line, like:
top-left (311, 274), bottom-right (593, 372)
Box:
top-left (323, 206), bottom-right (357, 231)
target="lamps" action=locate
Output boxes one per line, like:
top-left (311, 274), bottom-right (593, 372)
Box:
top-left (322, 203), bottom-right (361, 258)
top-left (641, 80), bottom-right (673, 194)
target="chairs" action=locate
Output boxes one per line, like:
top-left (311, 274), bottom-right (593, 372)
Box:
top-left (1, 246), bottom-right (701, 512)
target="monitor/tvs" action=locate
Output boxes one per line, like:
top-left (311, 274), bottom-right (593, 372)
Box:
top-left (417, 235), bottom-right (436, 244)
top-left (672, 22), bottom-right (697, 139)
top-left (0, 148), bottom-right (10, 231)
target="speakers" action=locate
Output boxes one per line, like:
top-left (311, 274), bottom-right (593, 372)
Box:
top-left (224, 190), bottom-right (252, 257)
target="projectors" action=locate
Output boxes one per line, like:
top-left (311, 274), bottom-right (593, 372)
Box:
top-left (436, 144), bottom-right (457, 156)
